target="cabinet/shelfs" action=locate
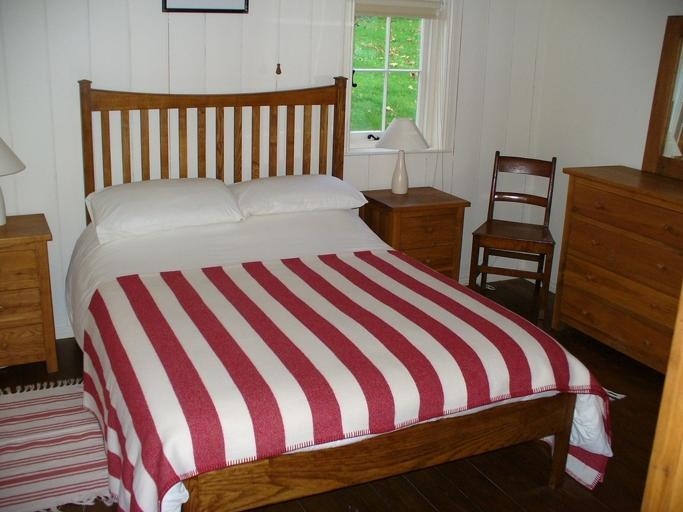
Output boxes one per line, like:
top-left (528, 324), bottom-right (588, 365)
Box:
top-left (551, 166), bottom-right (683, 374)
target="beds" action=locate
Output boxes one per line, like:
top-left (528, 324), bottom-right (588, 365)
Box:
top-left (66, 76), bottom-right (577, 512)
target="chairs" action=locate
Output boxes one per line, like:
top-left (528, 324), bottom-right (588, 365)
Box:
top-left (469, 151), bottom-right (557, 319)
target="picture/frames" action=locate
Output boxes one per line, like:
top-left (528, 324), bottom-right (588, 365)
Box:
top-left (641, 15), bottom-right (683, 180)
top-left (162, 0), bottom-right (249, 14)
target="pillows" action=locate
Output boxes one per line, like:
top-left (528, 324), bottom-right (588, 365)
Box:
top-left (227, 175), bottom-right (369, 217)
top-left (85, 178), bottom-right (245, 245)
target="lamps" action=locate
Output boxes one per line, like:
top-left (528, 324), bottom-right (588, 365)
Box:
top-left (0, 137), bottom-right (26, 226)
top-left (375, 117), bottom-right (430, 195)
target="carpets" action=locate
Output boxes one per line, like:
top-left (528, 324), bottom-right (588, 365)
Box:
top-left (0, 379), bottom-right (118, 512)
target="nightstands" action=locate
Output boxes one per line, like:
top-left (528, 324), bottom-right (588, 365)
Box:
top-left (359, 186), bottom-right (471, 283)
top-left (0, 213), bottom-right (59, 374)
